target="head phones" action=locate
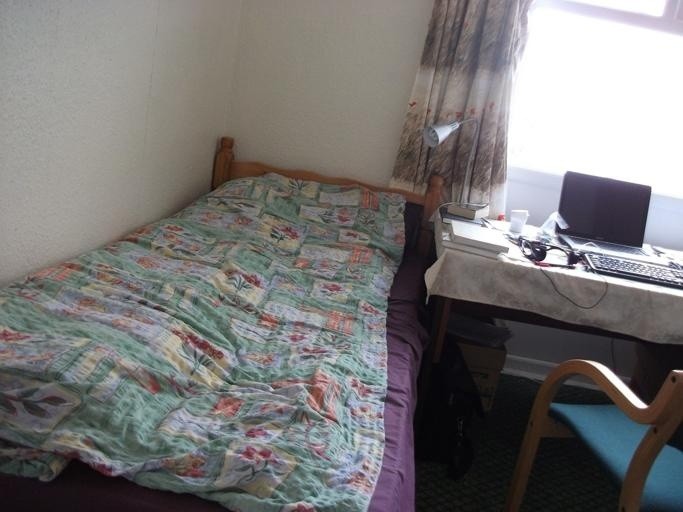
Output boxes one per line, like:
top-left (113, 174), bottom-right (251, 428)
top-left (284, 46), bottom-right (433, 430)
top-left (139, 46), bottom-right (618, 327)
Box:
top-left (521, 239), bottom-right (580, 265)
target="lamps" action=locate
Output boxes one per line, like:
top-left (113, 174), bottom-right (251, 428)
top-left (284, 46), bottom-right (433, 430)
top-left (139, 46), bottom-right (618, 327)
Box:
top-left (422, 119), bottom-right (480, 208)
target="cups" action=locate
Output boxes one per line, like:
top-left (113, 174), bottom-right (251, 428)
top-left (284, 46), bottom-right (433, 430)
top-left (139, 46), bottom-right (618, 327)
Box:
top-left (509, 209), bottom-right (529, 234)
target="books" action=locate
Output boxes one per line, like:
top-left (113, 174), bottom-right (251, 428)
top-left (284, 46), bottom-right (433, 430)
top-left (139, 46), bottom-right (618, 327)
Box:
top-left (441, 203), bottom-right (511, 261)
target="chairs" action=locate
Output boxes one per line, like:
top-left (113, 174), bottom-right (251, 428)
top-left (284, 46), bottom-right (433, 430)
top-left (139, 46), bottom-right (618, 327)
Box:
top-left (503, 359), bottom-right (682, 512)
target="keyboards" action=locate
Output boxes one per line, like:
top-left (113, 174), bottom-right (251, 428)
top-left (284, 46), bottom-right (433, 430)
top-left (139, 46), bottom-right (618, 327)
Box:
top-left (583, 253), bottom-right (683, 289)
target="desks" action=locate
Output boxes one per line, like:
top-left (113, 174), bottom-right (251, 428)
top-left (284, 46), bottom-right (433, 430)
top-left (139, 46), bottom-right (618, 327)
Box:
top-left (430, 210), bottom-right (682, 407)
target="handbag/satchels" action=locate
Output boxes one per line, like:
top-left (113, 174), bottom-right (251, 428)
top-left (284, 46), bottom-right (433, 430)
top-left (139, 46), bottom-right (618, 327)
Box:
top-left (413, 337), bottom-right (486, 464)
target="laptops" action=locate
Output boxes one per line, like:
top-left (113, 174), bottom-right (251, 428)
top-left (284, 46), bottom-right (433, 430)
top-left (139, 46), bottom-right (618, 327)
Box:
top-left (556, 171), bottom-right (669, 267)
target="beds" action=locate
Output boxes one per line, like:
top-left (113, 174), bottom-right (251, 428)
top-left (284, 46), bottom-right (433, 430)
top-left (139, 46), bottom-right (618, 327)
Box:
top-left (0, 136), bottom-right (444, 512)
